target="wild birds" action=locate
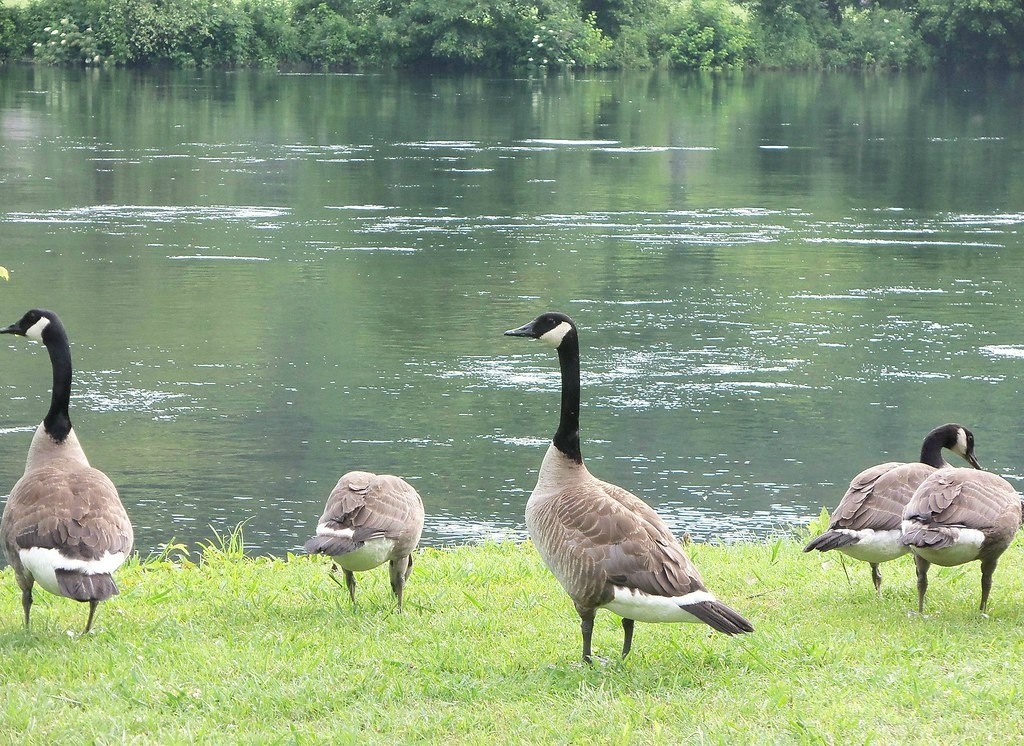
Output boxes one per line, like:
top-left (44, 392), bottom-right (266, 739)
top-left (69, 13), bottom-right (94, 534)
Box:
top-left (503, 313), bottom-right (755, 665)
top-left (902, 465), bottom-right (1024, 612)
top-left (303, 470), bottom-right (424, 612)
top-left (1, 308), bottom-right (136, 634)
top-left (802, 424), bottom-right (983, 592)
